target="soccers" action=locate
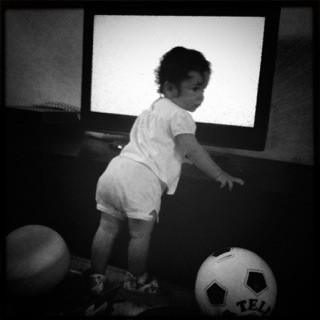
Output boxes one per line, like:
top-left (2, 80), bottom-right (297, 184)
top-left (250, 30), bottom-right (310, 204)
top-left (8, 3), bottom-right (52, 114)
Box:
top-left (195, 247), bottom-right (277, 320)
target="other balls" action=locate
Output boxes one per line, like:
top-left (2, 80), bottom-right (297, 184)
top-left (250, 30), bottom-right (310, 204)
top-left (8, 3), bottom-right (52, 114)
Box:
top-left (6, 225), bottom-right (71, 293)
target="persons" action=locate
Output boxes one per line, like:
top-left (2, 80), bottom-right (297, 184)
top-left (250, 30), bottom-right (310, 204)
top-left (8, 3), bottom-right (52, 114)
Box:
top-left (81, 45), bottom-right (246, 302)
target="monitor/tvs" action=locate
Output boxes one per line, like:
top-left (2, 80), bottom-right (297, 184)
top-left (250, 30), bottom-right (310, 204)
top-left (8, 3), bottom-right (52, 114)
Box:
top-left (80, 9), bottom-right (281, 151)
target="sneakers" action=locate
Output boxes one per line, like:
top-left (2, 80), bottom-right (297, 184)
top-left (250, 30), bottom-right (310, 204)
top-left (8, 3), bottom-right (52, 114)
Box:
top-left (87, 273), bottom-right (105, 295)
top-left (120, 271), bottom-right (161, 294)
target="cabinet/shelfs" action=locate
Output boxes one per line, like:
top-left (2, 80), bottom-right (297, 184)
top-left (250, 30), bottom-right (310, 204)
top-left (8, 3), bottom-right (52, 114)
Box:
top-left (2, 134), bottom-right (320, 306)
